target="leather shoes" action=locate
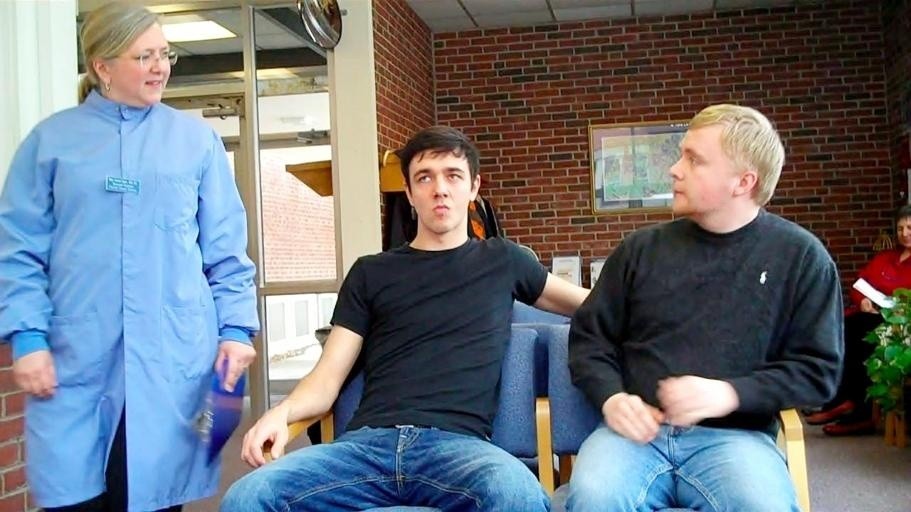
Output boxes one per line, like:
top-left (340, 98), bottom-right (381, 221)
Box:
top-left (822, 420), bottom-right (875, 438)
top-left (807, 400), bottom-right (857, 426)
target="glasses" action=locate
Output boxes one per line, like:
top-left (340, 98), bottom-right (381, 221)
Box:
top-left (103, 49), bottom-right (180, 71)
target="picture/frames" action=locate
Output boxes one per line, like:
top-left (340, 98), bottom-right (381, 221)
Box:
top-left (583, 112), bottom-right (695, 219)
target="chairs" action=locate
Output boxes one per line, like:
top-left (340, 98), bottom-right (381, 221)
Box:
top-left (254, 286), bottom-right (817, 512)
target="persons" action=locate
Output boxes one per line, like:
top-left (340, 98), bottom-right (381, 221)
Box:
top-left (565, 104), bottom-right (845, 512)
top-left (215, 125), bottom-right (593, 512)
top-left (0, 0), bottom-right (261, 512)
top-left (807, 204), bottom-right (911, 436)
